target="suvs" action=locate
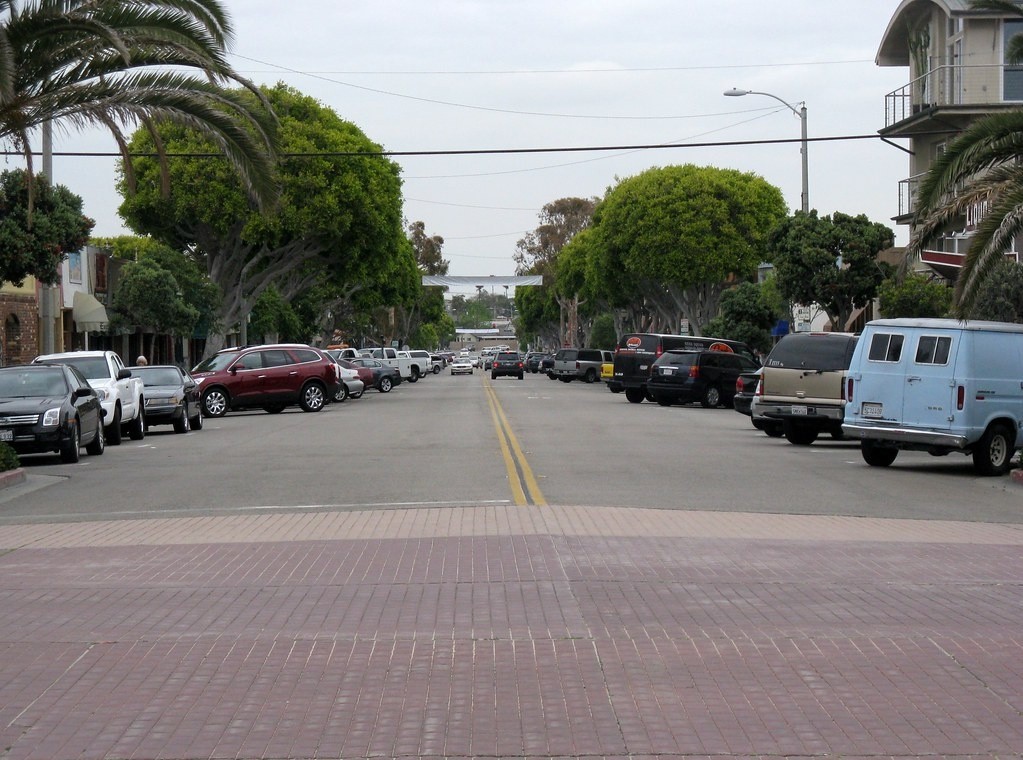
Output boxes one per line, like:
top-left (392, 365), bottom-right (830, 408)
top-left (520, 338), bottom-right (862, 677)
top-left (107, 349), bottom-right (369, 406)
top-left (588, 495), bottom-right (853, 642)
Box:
top-left (189, 344), bottom-right (336, 417)
top-left (646, 348), bottom-right (760, 410)
top-left (755, 332), bottom-right (862, 447)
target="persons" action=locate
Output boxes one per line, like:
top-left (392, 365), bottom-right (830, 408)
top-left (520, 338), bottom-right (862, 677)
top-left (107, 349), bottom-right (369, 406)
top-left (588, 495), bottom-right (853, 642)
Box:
top-left (136, 355), bottom-right (148, 366)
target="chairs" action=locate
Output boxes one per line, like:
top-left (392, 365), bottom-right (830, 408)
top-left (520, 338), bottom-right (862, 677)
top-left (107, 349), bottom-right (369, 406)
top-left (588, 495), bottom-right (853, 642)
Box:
top-left (47, 376), bottom-right (64, 395)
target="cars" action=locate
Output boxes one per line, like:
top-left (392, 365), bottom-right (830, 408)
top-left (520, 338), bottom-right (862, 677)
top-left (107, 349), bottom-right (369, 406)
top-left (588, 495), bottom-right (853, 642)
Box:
top-left (451, 344), bottom-right (511, 376)
top-left (126, 365), bottom-right (203, 434)
top-left (490, 350), bottom-right (523, 381)
top-left (0, 364), bottom-right (107, 463)
top-left (319, 344), bottom-right (455, 403)
top-left (29, 351), bottom-right (146, 446)
top-left (731, 364), bottom-right (786, 440)
top-left (518, 350), bottom-right (563, 382)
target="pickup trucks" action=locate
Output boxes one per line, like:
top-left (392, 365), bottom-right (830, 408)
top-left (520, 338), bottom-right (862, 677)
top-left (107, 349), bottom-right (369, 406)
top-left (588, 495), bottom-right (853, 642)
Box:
top-left (600, 364), bottom-right (626, 394)
top-left (357, 348), bottom-right (428, 383)
top-left (554, 348), bottom-right (616, 385)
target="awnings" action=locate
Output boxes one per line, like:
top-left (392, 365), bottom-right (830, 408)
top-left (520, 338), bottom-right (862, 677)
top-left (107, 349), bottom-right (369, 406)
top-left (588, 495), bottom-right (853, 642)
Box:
top-left (73, 291), bottom-right (109, 333)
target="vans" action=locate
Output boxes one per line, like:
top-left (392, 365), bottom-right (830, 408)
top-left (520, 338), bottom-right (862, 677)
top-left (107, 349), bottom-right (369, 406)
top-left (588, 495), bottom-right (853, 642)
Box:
top-left (839, 318), bottom-right (1023, 479)
top-left (605, 332), bottom-right (764, 405)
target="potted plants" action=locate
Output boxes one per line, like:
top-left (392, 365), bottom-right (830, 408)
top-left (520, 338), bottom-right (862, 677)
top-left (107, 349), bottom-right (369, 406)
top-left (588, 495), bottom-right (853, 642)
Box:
top-left (904, 4), bottom-right (936, 115)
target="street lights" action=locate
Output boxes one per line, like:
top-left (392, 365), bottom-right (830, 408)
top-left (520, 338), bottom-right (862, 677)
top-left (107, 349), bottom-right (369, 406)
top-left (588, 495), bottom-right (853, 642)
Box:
top-left (724, 87), bottom-right (809, 213)
